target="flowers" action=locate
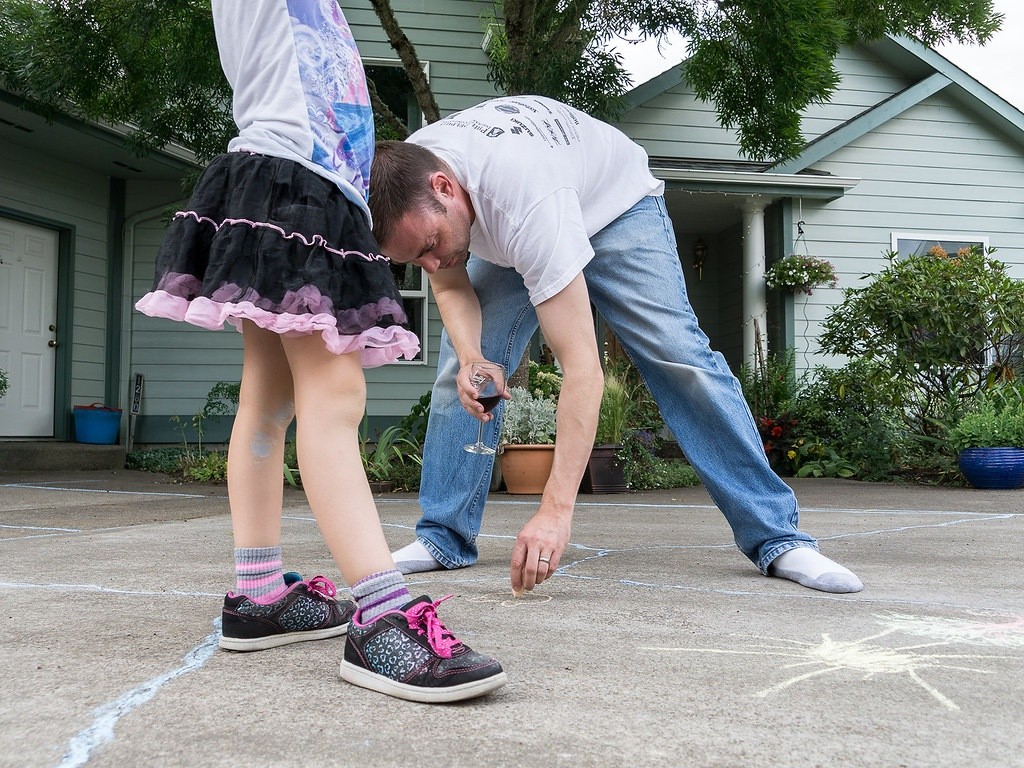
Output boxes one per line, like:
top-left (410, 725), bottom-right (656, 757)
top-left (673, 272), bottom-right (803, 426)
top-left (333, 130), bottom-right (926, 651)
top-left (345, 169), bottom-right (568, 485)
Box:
top-left (762, 254), bottom-right (840, 295)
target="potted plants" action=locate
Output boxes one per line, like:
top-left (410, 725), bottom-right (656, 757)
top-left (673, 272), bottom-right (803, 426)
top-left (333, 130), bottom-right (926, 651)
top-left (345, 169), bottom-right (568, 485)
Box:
top-left (589, 362), bottom-right (659, 494)
top-left (498, 385), bottom-right (558, 496)
top-left (943, 400), bottom-right (1024, 490)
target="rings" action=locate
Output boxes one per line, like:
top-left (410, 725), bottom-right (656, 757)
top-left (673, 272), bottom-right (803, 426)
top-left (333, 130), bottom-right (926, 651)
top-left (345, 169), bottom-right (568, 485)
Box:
top-left (538, 557), bottom-right (550, 564)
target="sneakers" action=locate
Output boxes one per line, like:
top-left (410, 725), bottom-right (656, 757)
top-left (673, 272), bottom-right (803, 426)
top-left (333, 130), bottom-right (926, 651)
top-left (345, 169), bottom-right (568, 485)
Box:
top-left (218, 572), bottom-right (358, 651)
top-left (340, 595), bottom-right (507, 703)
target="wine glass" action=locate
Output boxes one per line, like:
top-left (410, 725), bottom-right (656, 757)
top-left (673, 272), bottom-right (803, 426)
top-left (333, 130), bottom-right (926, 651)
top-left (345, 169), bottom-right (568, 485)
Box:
top-left (463, 361), bottom-right (507, 454)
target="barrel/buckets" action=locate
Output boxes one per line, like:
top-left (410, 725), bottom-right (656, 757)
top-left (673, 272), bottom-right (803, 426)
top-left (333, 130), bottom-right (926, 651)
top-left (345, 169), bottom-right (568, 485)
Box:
top-left (72, 404), bottom-right (124, 444)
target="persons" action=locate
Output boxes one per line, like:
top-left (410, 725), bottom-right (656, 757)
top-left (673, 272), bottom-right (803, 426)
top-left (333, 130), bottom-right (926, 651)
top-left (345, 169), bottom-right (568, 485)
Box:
top-left (371, 93), bottom-right (869, 594)
top-left (134, 0), bottom-right (505, 703)
